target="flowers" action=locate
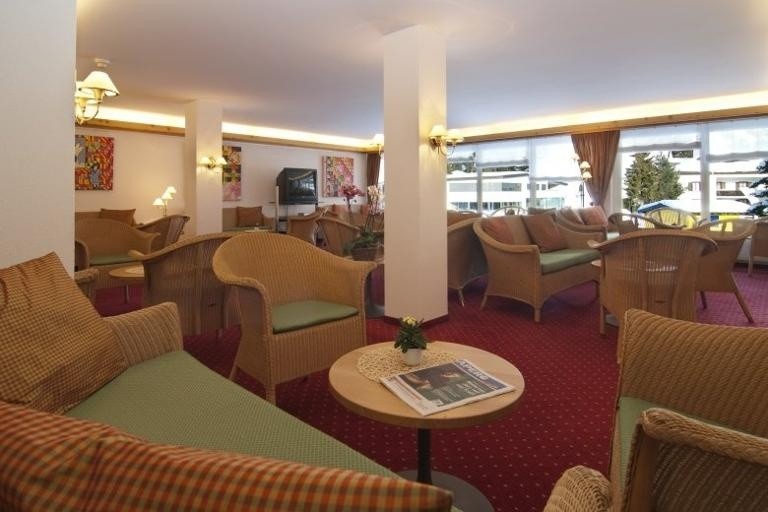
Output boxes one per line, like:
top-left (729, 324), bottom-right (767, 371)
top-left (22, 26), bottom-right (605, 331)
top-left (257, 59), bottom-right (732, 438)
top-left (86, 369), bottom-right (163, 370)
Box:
top-left (337, 183), bottom-right (366, 199)
top-left (367, 185), bottom-right (384, 200)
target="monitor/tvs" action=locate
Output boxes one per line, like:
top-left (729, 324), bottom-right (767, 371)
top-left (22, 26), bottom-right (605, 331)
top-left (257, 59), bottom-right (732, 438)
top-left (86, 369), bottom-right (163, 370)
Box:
top-left (276, 168), bottom-right (318, 205)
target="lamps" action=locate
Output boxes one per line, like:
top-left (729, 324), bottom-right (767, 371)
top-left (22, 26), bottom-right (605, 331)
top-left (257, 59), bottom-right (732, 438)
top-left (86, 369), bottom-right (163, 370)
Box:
top-left (75, 71), bottom-right (120, 125)
top-left (429, 124), bottom-right (464, 156)
top-left (373, 134), bottom-right (384, 155)
top-left (201, 156), bottom-right (228, 168)
top-left (571, 154), bottom-right (592, 181)
top-left (153, 186), bottom-right (176, 216)
